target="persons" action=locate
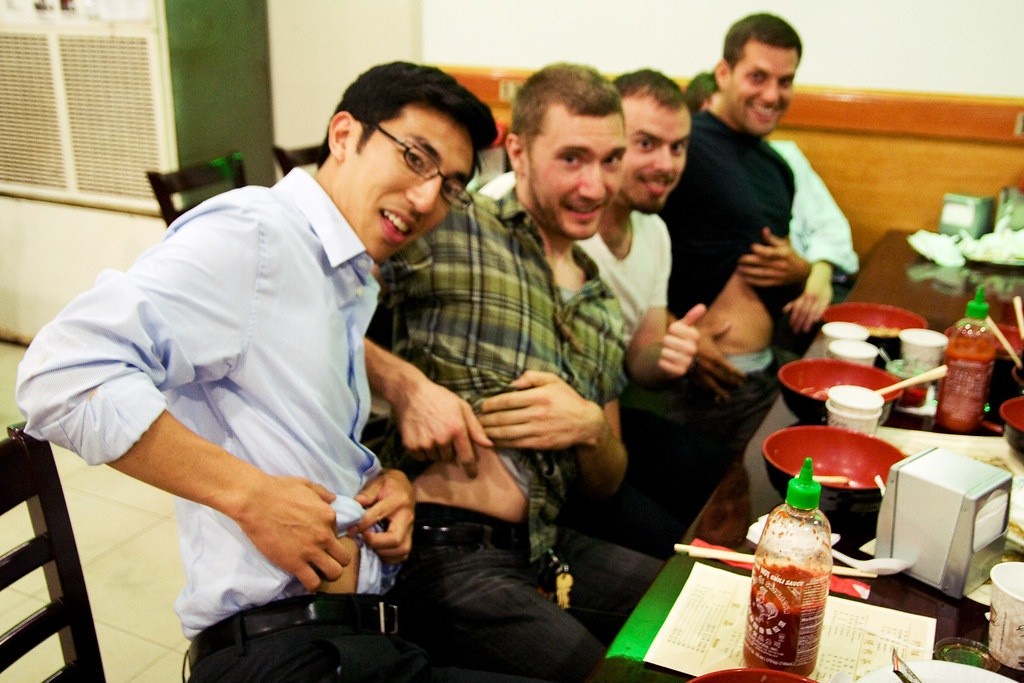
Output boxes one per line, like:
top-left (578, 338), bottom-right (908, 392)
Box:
top-left (362, 62), bottom-right (665, 683)
top-left (478, 13), bottom-right (859, 560)
top-left (14, 62), bottom-right (498, 683)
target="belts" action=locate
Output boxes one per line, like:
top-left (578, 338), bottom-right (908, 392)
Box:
top-left (189, 598), bottom-right (399, 671)
top-left (412, 522), bottom-right (530, 550)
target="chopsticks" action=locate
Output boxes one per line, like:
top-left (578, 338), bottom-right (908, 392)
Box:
top-left (857, 661), bottom-right (1019, 683)
top-left (985, 295), bottom-right (1024, 369)
top-left (674, 544), bottom-right (878, 578)
top-left (873, 365), bottom-right (948, 395)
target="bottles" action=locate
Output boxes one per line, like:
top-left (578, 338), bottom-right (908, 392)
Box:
top-left (744, 458), bottom-right (832, 677)
top-left (936, 284), bottom-right (998, 431)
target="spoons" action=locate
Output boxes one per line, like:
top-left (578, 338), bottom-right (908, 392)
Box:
top-left (831, 550), bottom-right (910, 574)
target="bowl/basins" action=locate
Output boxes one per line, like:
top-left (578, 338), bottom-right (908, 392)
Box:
top-left (1000, 396), bottom-right (1024, 456)
top-left (821, 301), bottom-right (928, 367)
top-left (762, 426), bottom-right (907, 528)
top-left (944, 323), bottom-right (1024, 374)
top-left (776, 357), bottom-right (902, 429)
top-left (1010, 362), bottom-right (1024, 395)
top-left (688, 668), bottom-right (820, 683)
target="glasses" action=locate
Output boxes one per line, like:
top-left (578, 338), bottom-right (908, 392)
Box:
top-left (374, 124), bottom-right (473, 210)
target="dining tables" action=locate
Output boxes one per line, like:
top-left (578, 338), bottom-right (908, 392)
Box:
top-left (584, 229), bottom-right (1024, 683)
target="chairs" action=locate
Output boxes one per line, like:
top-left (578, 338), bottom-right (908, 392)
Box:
top-left (145, 142), bottom-right (321, 229)
top-left (0, 420), bottom-right (112, 683)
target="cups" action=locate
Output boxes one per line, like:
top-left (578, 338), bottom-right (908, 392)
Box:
top-left (898, 328), bottom-right (947, 378)
top-left (824, 387), bottom-right (883, 437)
top-left (822, 322), bottom-right (870, 358)
top-left (988, 562), bottom-right (1024, 671)
top-left (828, 339), bottom-right (878, 366)
